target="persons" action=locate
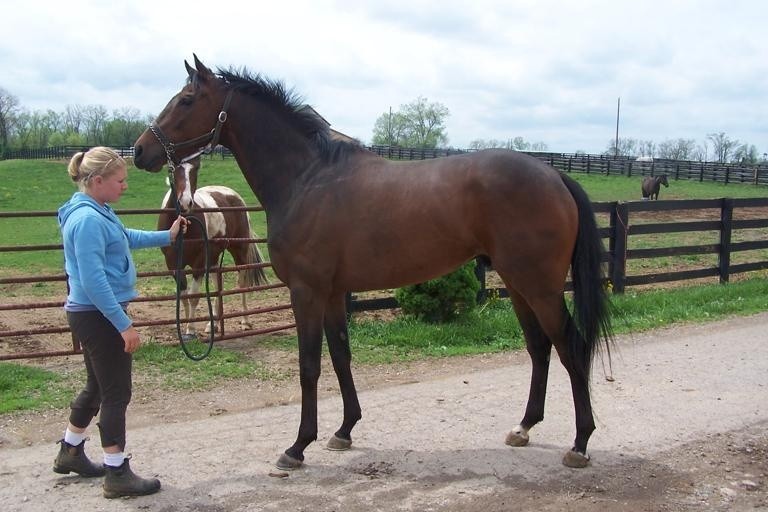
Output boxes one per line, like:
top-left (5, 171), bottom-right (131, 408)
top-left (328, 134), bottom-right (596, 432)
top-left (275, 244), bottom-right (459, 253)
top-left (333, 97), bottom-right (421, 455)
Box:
top-left (52, 147), bottom-right (193, 498)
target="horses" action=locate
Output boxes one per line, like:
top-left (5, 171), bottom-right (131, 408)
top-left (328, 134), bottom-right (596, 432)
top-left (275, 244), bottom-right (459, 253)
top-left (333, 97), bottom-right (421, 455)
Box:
top-left (133, 52), bottom-right (610, 470)
top-left (158, 148), bottom-right (271, 335)
top-left (642, 173), bottom-right (669, 201)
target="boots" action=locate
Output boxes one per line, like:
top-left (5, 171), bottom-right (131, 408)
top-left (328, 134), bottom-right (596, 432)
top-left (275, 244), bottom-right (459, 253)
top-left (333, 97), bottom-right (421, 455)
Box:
top-left (53, 438), bottom-right (106, 477)
top-left (103, 457), bottom-right (161, 498)
